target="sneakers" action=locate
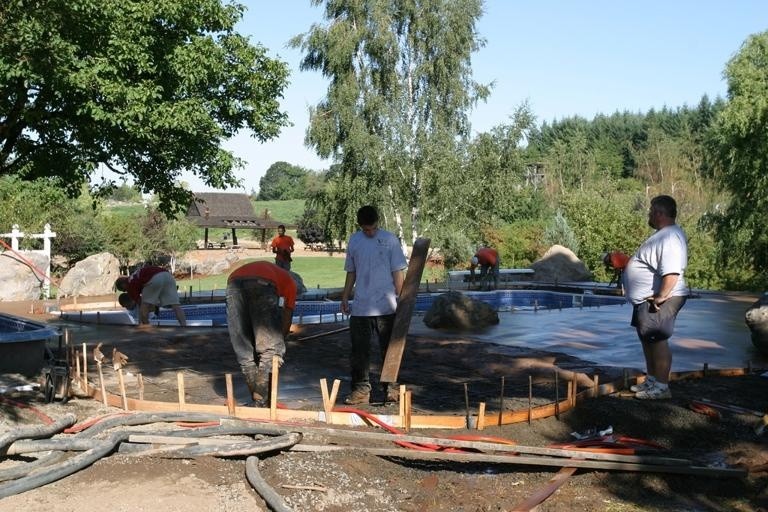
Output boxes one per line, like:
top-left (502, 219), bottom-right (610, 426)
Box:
top-left (248, 399), bottom-right (270, 408)
top-left (631, 377), bottom-right (655, 392)
top-left (635, 382), bottom-right (671, 399)
top-left (385, 390), bottom-right (399, 402)
top-left (344, 391), bottom-right (370, 404)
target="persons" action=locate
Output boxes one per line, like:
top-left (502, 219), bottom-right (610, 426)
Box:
top-left (339, 204), bottom-right (409, 404)
top-left (469, 247), bottom-right (502, 289)
top-left (622, 195), bottom-right (691, 401)
top-left (115, 265), bottom-right (188, 326)
top-left (225, 260), bottom-right (308, 407)
top-left (270, 224), bottom-right (295, 270)
top-left (117, 292), bottom-right (161, 324)
top-left (601, 251), bottom-right (631, 298)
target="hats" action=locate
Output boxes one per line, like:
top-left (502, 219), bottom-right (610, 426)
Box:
top-left (472, 257), bottom-right (478, 265)
